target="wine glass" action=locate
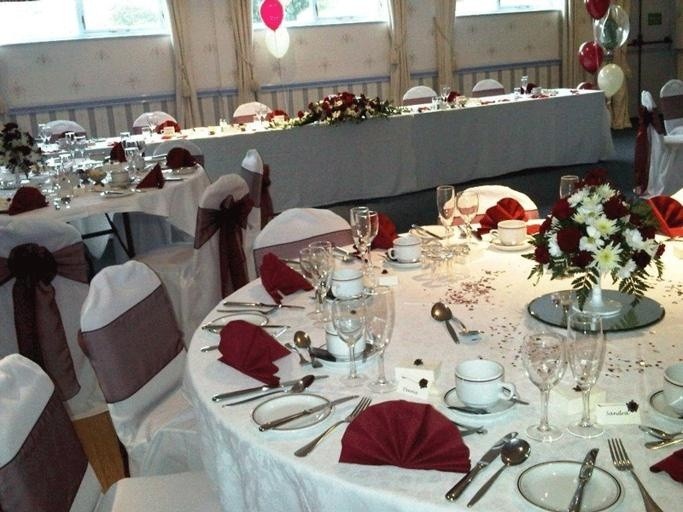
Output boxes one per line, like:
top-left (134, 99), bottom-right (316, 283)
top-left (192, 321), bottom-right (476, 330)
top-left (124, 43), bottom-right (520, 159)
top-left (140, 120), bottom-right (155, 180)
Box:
top-left (350, 206), bottom-right (371, 268)
top-left (125, 147), bottom-right (140, 181)
top-left (456, 191), bottom-right (479, 246)
top-left (559, 176), bottom-right (579, 199)
top-left (440, 85), bottom-right (448, 105)
top-left (362, 285), bottom-right (397, 393)
top-left (38, 123), bottom-right (53, 148)
top-left (521, 79), bottom-right (528, 96)
top-left (299, 240), bottom-right (334, 321)
top-left (56, 132), bottom-right (86, 161)
top-left (356, 210), bottom-right (379, 273)
top-left (125, 141), bottom-right (136, 161)
top-left (523, 332), bottom-right (567, 444)
top-left (522, 75), bottom-right (528, 79)
top-left (436, 185), bottom-right (455, 241)
top-left (443, 87), bottom-right (451, 106)
top-left (332, 294), bottom-right (368, 388)
top-left (567, 313), bottom-right (606, 440)
top-left (255, 104), bottom-right (268, 126)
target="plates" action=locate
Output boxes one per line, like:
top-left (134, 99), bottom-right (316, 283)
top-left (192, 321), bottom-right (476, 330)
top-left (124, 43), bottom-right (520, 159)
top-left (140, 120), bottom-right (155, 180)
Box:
top-left (409, 225), bottom-right (454, 240)
top-left (444, 388), bottom-right (519, 417)
top-left (648, 390), bottom-right (683, 422)
top-left (384, 259), bottom-right (423, 268)
top-left (318, 343), bottom-right (378, 365)
top-left (208, 313), bottom-right (269, 336)
top-left (491, 239), bottom-right (532, 251)
top-left (101, 190), bottom-right (134, 197)
top-left (517, 461), bottom-right (624, 512)
top-left (253, 394), bottom-right (333, 432)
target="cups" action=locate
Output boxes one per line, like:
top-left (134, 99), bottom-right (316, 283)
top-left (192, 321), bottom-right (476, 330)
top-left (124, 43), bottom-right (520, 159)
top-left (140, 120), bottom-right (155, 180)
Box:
top-left (387, 237), bottom-right (422, 263)
top-left (326, 323), bottom-right (365, 358)
top-left (142, 126), bottom-right (150, 137)
top-left (431, 97), bottom-right (438, 109)
top-left (663, 363), bottom-right (683, 413)
top-left (120, 132), bottom-right (131, 143)
top-left (490, 220), bottom-right (527, 246)
top-left (110, 171), bottom-right (129, 183)
top-left (454, 359), bottom-right (515, 408)
top-left (332, 270), bottom-right (365, 299)
top-left (514, 87), bottom-right (521, 99)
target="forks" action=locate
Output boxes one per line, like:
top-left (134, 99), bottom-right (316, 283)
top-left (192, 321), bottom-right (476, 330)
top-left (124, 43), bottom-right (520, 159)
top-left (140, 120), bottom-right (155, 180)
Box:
top-left (284, 342), bottom-right (311, 367)
top-left (294, 396), bottom-right (373, 457)
top-left (608, 438), bottom-right (663, 511)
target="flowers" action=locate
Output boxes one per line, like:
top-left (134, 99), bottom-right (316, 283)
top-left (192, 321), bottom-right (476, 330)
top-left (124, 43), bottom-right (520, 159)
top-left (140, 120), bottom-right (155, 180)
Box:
top-left (521, 168), bottom-right (667, 305)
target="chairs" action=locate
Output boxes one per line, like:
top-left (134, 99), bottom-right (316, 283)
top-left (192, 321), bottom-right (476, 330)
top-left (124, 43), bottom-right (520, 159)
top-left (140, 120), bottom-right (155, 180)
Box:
top-left (1, 351), bottom-right (239, 512)
top-left (447, 182), bottom-right (540, 223)
top-left (76, 260), bottom-right (217, 478)
top-left (251, 208), bottom-right (362, 279)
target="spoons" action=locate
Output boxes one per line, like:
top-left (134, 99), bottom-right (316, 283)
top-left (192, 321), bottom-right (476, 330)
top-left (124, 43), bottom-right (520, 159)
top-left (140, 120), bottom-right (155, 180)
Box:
top-left (465, 437), bottom-right (532, 507)
top-left (431, 302), bottom-right (459, 344)
top-left (638, 424), bottom-right (683, 440)
top-left (452, 315), bottom-right (484, 336)
top-left (217, 305), bottom-right (281, 314)
top-left (222, 375), bottom-right (315, 407)
top-left (61, 197), bottom-right (72, 210)
top-left (294, 330), bottom-right (324, 369)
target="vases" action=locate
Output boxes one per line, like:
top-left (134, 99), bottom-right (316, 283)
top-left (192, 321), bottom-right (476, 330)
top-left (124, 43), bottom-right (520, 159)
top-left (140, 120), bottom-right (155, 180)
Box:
top-left (572, 258), bottom-right (622, 316)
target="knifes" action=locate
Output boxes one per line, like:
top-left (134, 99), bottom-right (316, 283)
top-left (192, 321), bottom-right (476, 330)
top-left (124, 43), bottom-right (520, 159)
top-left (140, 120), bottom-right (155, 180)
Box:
top-left (52, 201), bottom-right (60, 211)
top-left (569, 447), bottom-right (600, 512)
top-left (224, 301), bottom-right (305, 310)
top-left (259, 395), bottom-right (359, 431)
top-left (444, 431), bottom-right (519, 501)
top-left (212, 375), bottom-right (331, 402)
top-left (411, 223), bottom-right (443, 240)
top-left (201, 324), bottom-right (291, 329)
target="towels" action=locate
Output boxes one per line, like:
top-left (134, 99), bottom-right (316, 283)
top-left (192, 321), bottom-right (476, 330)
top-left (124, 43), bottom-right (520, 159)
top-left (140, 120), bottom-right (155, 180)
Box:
top-left (212, 318), bottom-right (294, 388)
top-left (336, 397), bottom-right (474, 476)
top-left (255, 247), bottom-right (314, 305)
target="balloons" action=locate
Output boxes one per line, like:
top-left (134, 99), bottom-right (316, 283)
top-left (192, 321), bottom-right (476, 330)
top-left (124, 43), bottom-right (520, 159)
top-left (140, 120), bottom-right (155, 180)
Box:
top-left (577, 0), bottom-right (631, 101)
top-left (266, 28), bottom-right (290, 61)
top-left (260, 1), bottom-right (283, 30)
